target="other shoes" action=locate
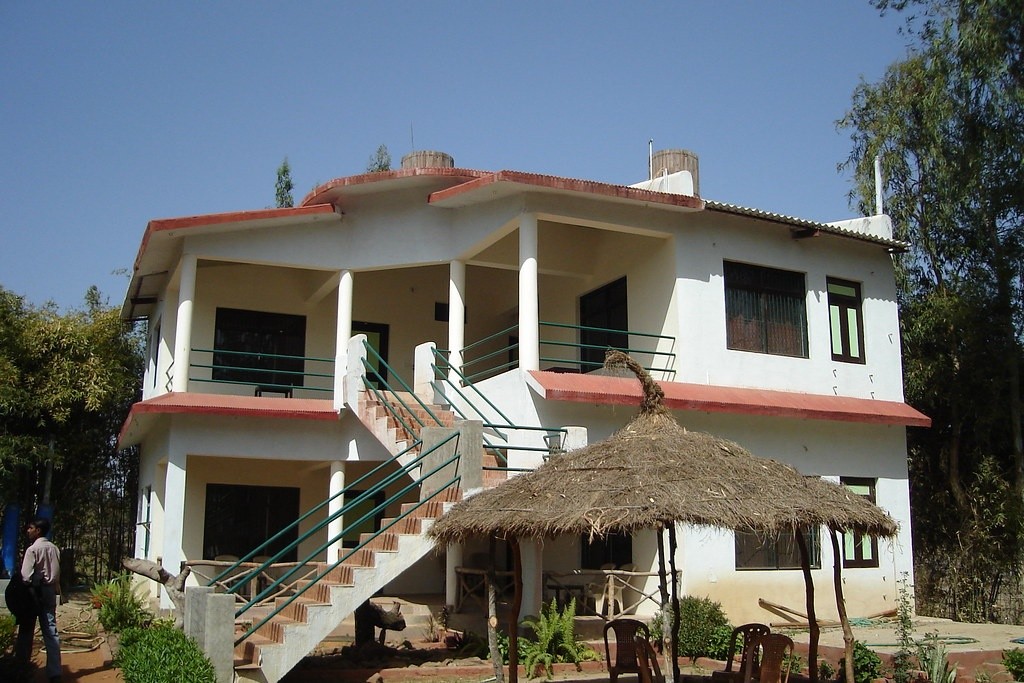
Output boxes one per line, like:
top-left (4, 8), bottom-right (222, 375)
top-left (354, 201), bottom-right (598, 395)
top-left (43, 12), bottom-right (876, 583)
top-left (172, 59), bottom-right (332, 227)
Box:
top-left (50, 675), bottom-right (62, 683)
top-left (7, 657), bottom-right (16, 662)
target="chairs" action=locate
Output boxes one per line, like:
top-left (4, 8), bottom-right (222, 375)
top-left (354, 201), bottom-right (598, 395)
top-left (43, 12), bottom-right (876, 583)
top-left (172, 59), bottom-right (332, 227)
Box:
top-left (253, 556), bottom-right (279, 594)
top-left (215, 555), bottom-right (239, 592)
top-left (603, 619), bottom-right (653, 683)
top-left (634, 636), bottom-right (664, 683)
top-left (584, 564), bottom-right (637, 617)
top-left (439, 553), bottom-right (446, 596)
top-left (713, 624), bottom-right (770, 683)
top-left (467, 553), bottom-right (491, 597)
top-left (745, 634), bottom-right (795, 683)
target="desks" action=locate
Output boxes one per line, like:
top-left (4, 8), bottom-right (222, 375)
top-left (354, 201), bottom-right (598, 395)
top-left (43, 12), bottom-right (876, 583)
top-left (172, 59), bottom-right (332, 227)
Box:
top-left (230, 576), bottom-right (267, 602)
top-left (548, 574), bottom-right (585, 616)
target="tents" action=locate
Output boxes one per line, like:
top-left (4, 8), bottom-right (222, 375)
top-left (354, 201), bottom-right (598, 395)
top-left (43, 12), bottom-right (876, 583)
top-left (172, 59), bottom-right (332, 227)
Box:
top-left (426, 347), bottom-right (898, 683)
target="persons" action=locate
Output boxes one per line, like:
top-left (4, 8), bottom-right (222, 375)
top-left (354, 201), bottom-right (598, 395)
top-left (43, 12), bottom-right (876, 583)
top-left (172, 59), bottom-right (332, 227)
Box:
top-left (10, 517), bottom-right (63, 683)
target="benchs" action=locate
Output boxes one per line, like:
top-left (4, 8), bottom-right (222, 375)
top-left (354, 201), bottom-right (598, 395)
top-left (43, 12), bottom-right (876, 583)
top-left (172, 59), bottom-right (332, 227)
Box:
top-left (255, 385), bottom-right (293, 398)
